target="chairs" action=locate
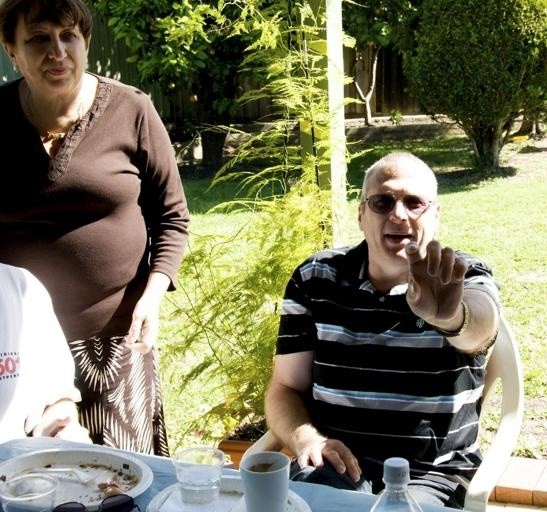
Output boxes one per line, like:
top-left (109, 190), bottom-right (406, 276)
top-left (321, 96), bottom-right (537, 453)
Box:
top-left (238, 299), bottom-right (525, 511)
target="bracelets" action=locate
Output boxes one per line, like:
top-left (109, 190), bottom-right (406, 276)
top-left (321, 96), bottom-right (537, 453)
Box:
top-left (0, 2), bottom-right (190, 461)
top-left (431, 298), bottom-right (471, 339)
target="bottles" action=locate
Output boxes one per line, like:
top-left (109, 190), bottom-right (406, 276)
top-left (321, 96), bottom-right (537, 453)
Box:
top-left (366, 457), bottom-right (423, 512)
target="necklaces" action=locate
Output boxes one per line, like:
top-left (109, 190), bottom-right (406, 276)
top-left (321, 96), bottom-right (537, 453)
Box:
top-left (24, 83), bottom-right (66, 144)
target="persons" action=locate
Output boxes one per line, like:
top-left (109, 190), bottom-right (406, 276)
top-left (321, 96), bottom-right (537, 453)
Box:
top-left (0, 258), bottom-right (96, 452)
top-left (259, 149), bottom-right (504, 508)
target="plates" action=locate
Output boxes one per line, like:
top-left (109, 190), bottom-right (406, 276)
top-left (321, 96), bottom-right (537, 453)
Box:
top-left (0, 446), bottom-right (156, 512)
top-left (143, 472), bottom-right (313, 512)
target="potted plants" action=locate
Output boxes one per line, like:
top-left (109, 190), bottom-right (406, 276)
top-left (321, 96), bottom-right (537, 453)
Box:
top-left (168, 43), bottom-right (348, 469)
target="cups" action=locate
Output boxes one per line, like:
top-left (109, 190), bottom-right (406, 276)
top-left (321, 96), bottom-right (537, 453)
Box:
top-left (0, 471), bottom-right (60, 512)
top-left (238, 449), bottom-right (293, 512)
top-left (170, 445), bottom-right (226, 510)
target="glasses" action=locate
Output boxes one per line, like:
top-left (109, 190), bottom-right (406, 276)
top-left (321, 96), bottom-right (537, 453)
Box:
top-left (365, 193), bottom-right (435, 216)
